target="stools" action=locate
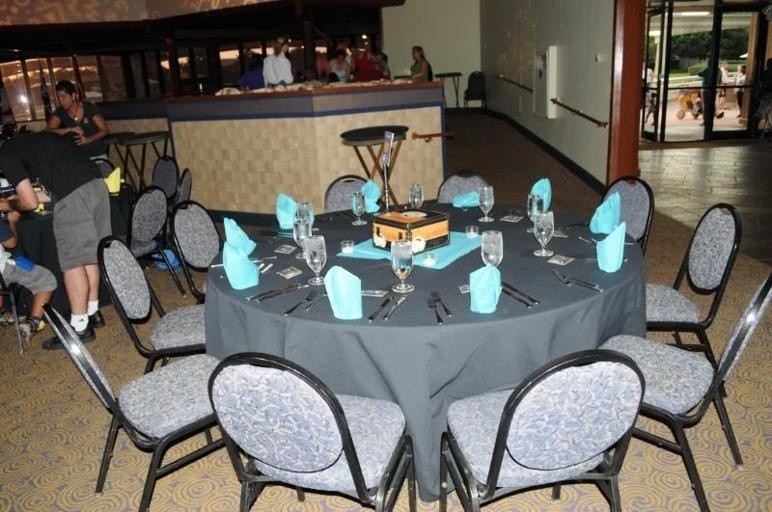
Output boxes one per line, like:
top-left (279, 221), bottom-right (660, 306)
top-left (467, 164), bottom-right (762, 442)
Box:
top-left (434, 73), bottom-right (462, 109)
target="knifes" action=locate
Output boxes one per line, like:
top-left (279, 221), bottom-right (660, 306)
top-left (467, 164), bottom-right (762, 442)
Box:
top-left (365, 294), bottom-right (409, 322)
top-left (247, 285), bottom-right (298, 303)
top-left (572, 278), bottom-right (604, 294)
top-left (260, 230), bottom-right (293, 238)
top-left (502, 281), bottom-right (541, 309)
top-left (305, 292), bottom-right (327, 312)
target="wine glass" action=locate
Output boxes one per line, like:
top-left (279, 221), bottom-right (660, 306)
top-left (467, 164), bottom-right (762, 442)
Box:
top-left (391, 240), bottom-right (415, 294)
top-left (292, 199), bottom-right (327, 286)
top-left (527, 193), bottom-right (555, 257)
top-left (477, 184), bottom-right (495, 223)
top-left (350, 192), bottom-right (367, 225)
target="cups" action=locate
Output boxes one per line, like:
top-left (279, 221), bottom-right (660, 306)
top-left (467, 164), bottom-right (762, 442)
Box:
top-left (340, 240), bottom-right (355, 254)
top-left (465, 226), bottom-right (478, 238)
top-left (409, 183), bottom-right (424, 208)
top-left (481, 230), bottom-right (504, 267)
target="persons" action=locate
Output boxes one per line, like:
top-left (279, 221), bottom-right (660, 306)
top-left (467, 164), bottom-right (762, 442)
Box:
top-left (641, 53), bottom-right (772, 141)
top-left (407, 46), bottom-right (433, 82)
top-left (0, 208), bottom-right (59, 340)
top-left (238, 32), bottom-right (391, 91)
top-left (0, 127), bottom-right (114, 351)
top-left (41, 80), bottom-right (111, 147)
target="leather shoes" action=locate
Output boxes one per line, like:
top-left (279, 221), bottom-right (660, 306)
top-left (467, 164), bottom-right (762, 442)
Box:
top-left (42, 310), bottom-right (105, 350)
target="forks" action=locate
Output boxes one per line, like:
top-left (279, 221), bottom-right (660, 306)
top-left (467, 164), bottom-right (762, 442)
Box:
top-left (283, 289), bottom-right (318, 317)
top-left (427, 291), bottom-right (453, 324)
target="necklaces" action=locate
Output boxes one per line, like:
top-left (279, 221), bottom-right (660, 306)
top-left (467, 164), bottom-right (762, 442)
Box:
top-left (64, 100), bottom-right (82, 122)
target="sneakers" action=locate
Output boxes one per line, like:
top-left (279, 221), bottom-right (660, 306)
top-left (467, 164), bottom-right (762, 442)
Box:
top-left (0, 306), bottom-right (36, 350)
